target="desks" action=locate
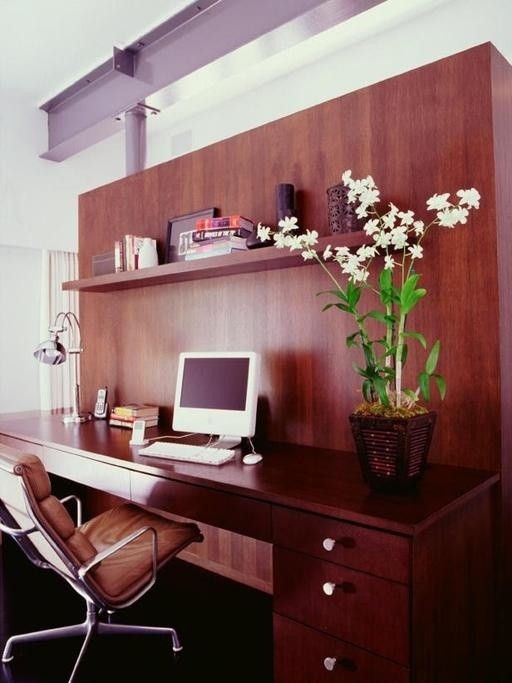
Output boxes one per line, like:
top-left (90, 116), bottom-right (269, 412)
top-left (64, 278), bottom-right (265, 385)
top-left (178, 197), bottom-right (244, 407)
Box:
top-left (2, 409), bottom-right (498, 682)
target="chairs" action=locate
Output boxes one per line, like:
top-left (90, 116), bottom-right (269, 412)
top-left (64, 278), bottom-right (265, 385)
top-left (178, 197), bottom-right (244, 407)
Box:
top-left (0, 442), bottom-right (208, 680)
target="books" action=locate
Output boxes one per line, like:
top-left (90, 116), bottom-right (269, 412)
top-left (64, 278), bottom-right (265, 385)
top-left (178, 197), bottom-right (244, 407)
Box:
top-left (109, 412), bottom-right (159, 422)
top-left (113, 401), bottom-right (159, 416)
top-left (112, 213), bottom-right (256, 274)
top-left (109, 419), bottom-right (159, 428)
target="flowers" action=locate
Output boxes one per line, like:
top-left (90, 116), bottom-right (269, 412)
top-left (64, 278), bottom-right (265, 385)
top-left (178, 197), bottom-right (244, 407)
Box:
top-left (254, 164), bottom-right (484, 410)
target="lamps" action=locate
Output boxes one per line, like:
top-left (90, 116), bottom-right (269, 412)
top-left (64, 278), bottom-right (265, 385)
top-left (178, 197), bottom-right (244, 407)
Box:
top-left (33, 310), bottom-right (95, 424)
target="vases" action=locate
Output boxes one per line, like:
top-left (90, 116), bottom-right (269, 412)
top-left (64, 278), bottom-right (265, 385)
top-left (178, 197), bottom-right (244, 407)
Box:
top-left (347, 391), bottom-right (436, 486)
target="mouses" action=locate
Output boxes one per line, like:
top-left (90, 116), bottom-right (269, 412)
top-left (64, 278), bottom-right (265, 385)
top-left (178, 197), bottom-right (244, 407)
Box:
top-left (242, 452), bottom-right (263, 465)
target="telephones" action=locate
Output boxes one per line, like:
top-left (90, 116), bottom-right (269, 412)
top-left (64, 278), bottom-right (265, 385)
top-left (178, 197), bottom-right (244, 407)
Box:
top-left (94, 385), bottom-right (108, 419)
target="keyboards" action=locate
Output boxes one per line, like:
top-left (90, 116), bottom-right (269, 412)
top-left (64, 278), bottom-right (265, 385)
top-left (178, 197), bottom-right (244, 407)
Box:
top-left (138, 440), bottom-right (237, 466)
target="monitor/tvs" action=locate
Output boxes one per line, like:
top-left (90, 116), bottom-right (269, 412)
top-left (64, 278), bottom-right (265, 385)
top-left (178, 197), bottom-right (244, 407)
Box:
top-left (171, 351), bottom-right (261, 448)
